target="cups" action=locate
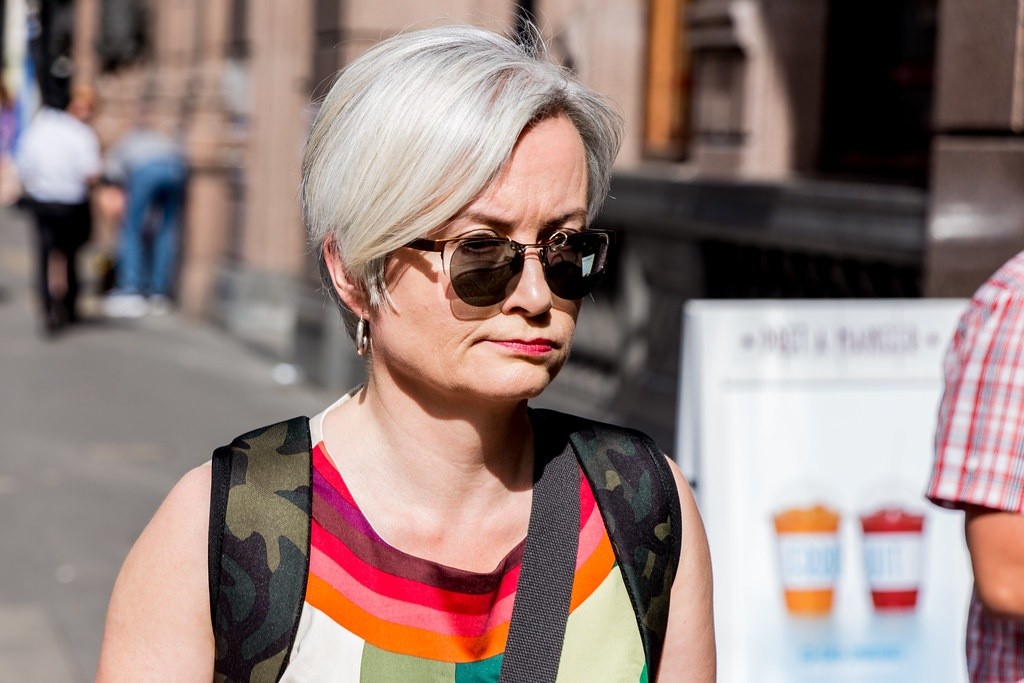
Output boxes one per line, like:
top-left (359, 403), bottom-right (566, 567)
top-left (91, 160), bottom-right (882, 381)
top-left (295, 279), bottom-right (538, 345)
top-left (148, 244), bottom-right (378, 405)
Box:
top-left (859, 513), bottom-right (924, 612)
top-left (774, 512), bottom-right (842, 619)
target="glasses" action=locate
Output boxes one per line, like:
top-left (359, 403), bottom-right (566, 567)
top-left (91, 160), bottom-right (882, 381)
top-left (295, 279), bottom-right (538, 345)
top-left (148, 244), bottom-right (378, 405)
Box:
top-left (389, 228), bottom-right (613, 305)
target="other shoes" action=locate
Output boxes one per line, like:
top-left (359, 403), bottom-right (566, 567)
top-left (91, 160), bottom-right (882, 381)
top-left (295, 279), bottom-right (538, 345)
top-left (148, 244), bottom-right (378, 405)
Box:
top-left (101, 291), bottom-right (166, 318)
top-left (42, 295), bottom-right (81, 329)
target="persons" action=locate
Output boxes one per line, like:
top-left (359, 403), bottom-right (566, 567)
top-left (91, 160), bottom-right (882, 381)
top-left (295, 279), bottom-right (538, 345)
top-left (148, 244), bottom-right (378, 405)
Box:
top-left (101, 132), bottom-right (188, 317)
top-left (927, 252), bottom-right (1024, 683)
top-left (96, 25), bottom-right (717, 683)
top-left (16, 84), bottom-right (102, 336)
